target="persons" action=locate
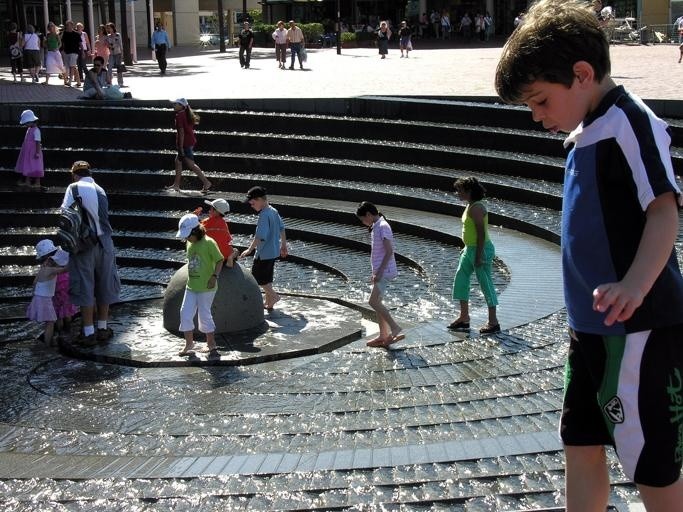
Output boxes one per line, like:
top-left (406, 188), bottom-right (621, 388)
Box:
top-left (5, 19), bottom-right (127, 89)
top-left (354, 199), bottom-right (405, 348)
top-left (240, 186), bottom-right (288, 309)
top-left (272, 20), bottom-right (288, 70)
top-left (200, 198), bottom-right (239, 268)
top-left (163, 97), bottom-right (212, 193)
top-left (176, 214), bottom-right (225, 357)
top-left (287, 20), bottom-right (306, 71)
top-left (52, 272), bottom-right (77, 331)
top-left (25, 239), bottom-right (68, 349)
top-left (493, 0), bottom-right (683, 512)
top-left (514, 12), bottom-right (525, 27)
top-left (445, 175), bottom-right (500, 334)
top-left (674, 16), bottom-right (683, 24)
top-left (14, 109), bottom-right (44, 190)
top-left (376, 19), bottom-right (412, 59)
top-left (600, 5), bottom-right (631, 27)
top-left (239, 22), bottom-right (254, 70)
top-left (61, 160), bottom-right (122, 346)
top-left (341, 18), bottom-right (373, 32)
top-left (420, 8), bottom-right (452, 40)
top-left (148, 21), bottom-right (171, 76)
top-left (83, 55), bottom-right (134, 102)
top-left (457, 11), bottom-right (494, 44)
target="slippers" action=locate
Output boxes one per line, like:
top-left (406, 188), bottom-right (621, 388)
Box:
top-left (367, 335), bottom-right (405, 346)
top-left (480, 324), bottom-right (499, 333)
top-left (447, 321), bottom-right (469, 329)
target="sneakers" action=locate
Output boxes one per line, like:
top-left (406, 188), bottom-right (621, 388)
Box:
top-left (82, 328), bottom-right (113, 345)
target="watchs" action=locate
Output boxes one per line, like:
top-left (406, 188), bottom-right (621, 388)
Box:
top-left (212, 274), bottom-right (220, 279)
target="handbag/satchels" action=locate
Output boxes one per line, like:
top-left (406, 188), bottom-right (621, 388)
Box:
top-left (10, 44), bottom-right (22, 59)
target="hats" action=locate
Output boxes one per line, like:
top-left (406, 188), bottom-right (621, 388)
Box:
top-left (170, 98), bottom-right (188, 106)
top-left (35, 239), bottom-right (69, 266)
top-left (204, 198), bottom-right (230, 215)
top-left (20, 110), bottom-right (38, 124)
top-left (176, 214), bottom-right (198, 238)
top-left (72, 161), bottom-right (89, 171)
top-left (243, 186), bottom-right (264, 203)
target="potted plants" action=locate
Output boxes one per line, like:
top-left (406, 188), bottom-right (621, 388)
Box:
top-left (339, 31), bottom-right (356, 48)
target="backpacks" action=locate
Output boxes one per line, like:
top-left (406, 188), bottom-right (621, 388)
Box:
top-left (57, 184), bottom-right (97, 254)
